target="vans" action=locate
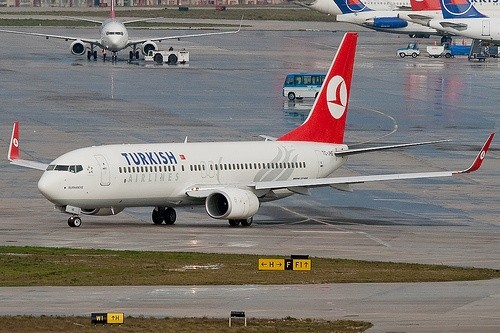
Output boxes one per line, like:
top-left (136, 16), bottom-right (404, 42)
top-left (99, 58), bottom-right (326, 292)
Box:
top-left (282, 72), bottom-right (327, 101)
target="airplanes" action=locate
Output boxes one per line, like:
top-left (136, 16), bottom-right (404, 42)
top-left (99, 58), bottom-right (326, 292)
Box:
top-left (7, 32), bottom-right (496, 227)
top-left (0, 0), bottom-right (243, 63)
top-left (291, 0), bottom-right (500, 57)
